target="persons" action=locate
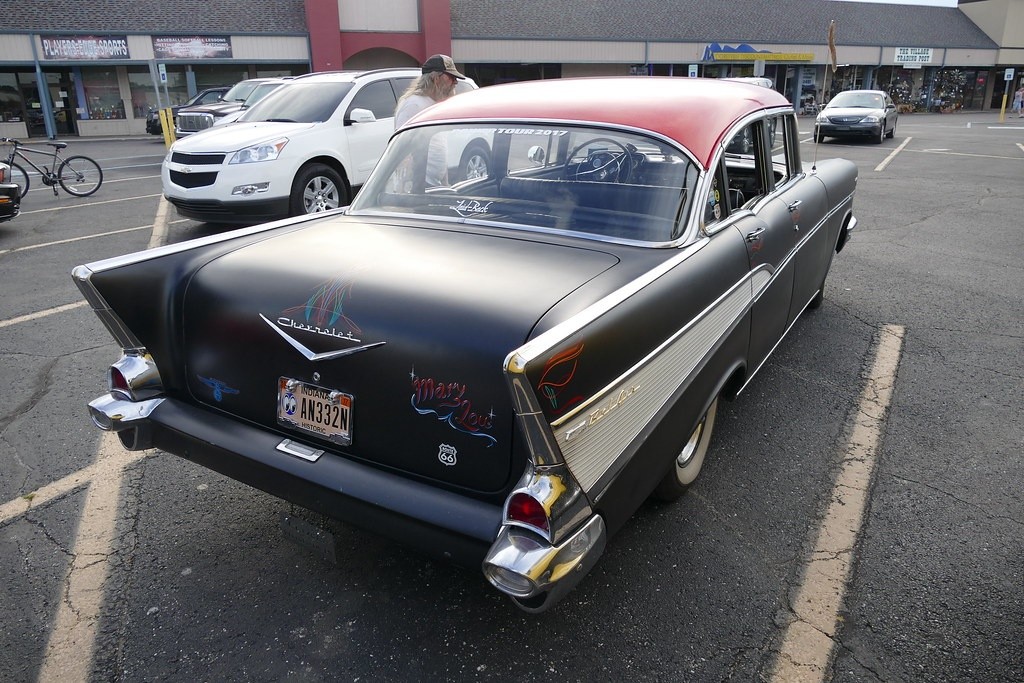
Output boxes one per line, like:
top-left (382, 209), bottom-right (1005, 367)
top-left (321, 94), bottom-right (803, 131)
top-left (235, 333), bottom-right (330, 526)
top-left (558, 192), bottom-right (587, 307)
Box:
top-left (1009, 87), bottom-right (1024, 118)
top-left (392, 53), bottom-right (466, 194)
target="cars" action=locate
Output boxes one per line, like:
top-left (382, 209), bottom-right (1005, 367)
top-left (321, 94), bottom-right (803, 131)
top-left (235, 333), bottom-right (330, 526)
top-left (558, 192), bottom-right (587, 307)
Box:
top-left (814, 90), bottom-right (898, 144)
top-left (71, 77), bottom-right (859, 614)
top-left (0, 162), bottom-right (22, 223)
top-left (146, 76), bottom-right (298, 137)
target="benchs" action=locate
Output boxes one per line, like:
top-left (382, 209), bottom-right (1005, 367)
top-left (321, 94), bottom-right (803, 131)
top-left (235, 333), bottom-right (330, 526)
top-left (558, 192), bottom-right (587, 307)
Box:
top-left (501, 176), bottom-right (688, 218)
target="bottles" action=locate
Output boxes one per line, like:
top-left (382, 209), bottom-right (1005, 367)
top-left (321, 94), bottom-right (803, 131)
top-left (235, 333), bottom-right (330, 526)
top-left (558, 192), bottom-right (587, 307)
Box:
top-left (88, 102), bottom-right (125, 119)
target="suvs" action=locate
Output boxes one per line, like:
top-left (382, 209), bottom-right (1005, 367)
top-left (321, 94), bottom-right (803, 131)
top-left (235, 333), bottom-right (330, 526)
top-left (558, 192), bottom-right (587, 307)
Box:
top-left (161, 68), bottom-right (480, 224)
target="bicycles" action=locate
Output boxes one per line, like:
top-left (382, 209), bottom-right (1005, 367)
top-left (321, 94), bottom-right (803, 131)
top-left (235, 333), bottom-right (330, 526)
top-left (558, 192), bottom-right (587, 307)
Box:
top-left (0, 137), bottom-right (103, 199)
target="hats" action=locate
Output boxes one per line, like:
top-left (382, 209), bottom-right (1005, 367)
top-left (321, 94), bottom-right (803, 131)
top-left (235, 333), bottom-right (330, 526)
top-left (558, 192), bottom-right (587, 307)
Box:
top-left (422, 54), bottom-right (466, 80)
